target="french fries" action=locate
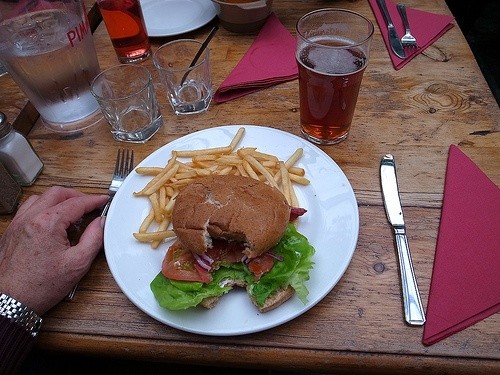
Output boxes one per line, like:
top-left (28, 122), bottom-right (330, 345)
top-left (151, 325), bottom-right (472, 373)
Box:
top-left (132, 127), bottom-right (311, 248)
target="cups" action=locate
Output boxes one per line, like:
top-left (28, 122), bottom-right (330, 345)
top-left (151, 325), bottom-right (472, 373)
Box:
top-left (0, 0), bottom-right (114, 135)
top-left (291, 7), bottom-right (375, 146)
top-left (95, 0), bottom-right (153, 65)
top-left (153, 39), bottom-right (213, 115)
top-left (211, 0), bottom-right (273, 33)
top-left (89, 62), bottom-right (164, 144)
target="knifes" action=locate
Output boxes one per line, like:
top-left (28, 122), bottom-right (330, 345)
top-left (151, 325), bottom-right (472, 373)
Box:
top-left (376, 0), bottom-right (406, 59)
top-left (379, 153), bottom-right (426, 326)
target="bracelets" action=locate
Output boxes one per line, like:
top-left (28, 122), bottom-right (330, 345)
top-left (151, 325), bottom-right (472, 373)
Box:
top-left (0, 293), bottom-right (43, 341)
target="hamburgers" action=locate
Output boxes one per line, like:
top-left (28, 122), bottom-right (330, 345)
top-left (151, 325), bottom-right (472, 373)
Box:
top-left (149, 176), bottom-right (316, 313)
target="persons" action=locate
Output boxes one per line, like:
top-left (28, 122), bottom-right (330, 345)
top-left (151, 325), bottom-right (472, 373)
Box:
top-left (0, 186), bottom-right (111, 375)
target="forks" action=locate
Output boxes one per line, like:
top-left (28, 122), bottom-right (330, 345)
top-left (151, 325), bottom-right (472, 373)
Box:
top-left (396, 2), bottom-right (419, 49)
top-left (64, 147), bottom-right (134, 297)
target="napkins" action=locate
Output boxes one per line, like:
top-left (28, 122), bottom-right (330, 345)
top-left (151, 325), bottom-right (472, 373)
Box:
top-left (210, 11), bottom-right (301, 103)
top-left (422, 143), bottom-right (500, 347)
top-left (368, 0), bottom-right (457, 70)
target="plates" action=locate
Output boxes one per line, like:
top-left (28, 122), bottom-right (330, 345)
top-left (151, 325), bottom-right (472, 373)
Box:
top-left (103, 124), bottom-right (360, 336)
top-left (139, 1), bottom-right (218, 37)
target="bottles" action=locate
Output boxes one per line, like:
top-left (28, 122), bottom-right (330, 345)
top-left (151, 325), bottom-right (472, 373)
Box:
top-left (0, 109), bottom-right (45, 219)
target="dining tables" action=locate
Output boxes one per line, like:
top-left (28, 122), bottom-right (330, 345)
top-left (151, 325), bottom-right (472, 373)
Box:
top-left (0, 0), bottom-right (500, 375)
